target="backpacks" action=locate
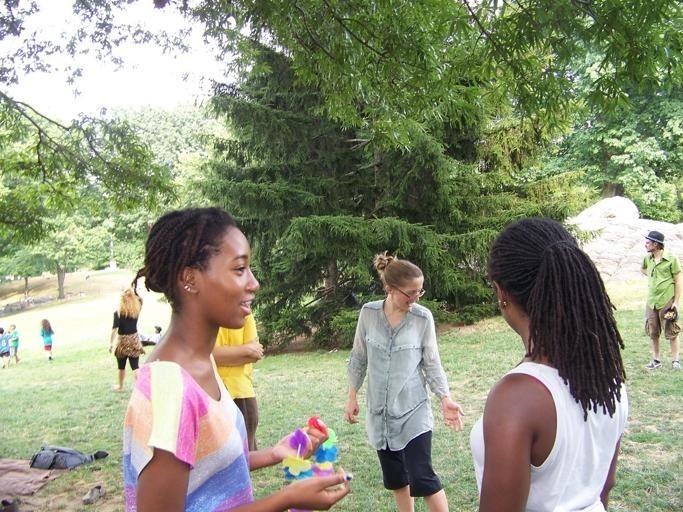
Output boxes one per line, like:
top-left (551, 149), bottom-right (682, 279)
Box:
top-left (28, 442), bottom-right (94, 473)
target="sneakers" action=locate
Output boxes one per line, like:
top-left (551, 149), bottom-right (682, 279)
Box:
top-left (672, 361), bottom-right (680, 371)
top-left (645, 361), bottom-right (662, 371)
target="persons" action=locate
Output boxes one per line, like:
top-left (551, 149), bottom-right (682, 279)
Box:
top-left (39, 319), bottom-right (57, 361)
top-left (212, 311), bottom-right (263, 452)
top-left (469, 216), bottom-right (630, 510)
top-left (640, 231), bottom-right (682, 371)
top-left (122, 207), bottom-right (354, 512)
top-left (343, 249), bottom-right (464, 511)
top-left (0, 327), bottom-right (10, 370)
top-left (108, 289), bottom-right (143, 393)
top-left (140, 326), bottom-right (162, 347)
top-left (8, 323), bottom-right (21, 364)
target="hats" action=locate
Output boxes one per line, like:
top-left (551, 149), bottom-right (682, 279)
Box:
top-left (643, 231), bottom-right (664, 245)
top-left (154, 326), bottom-right (161, 332)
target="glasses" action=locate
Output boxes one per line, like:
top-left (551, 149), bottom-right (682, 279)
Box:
top-left (390, 285), bottom-right (426, 302)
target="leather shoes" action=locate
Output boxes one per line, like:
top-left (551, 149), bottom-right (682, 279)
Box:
top-left (0, 499), bottom-right (19, 512)
top-left (83, 485), bottom-right (107, 505)
